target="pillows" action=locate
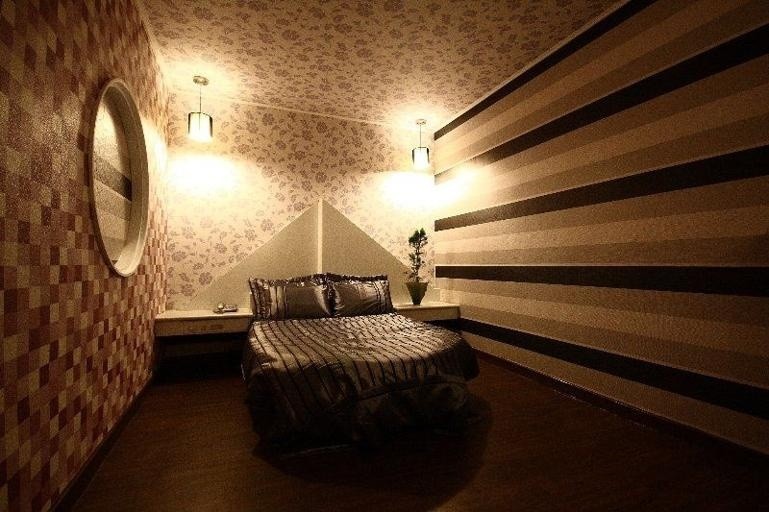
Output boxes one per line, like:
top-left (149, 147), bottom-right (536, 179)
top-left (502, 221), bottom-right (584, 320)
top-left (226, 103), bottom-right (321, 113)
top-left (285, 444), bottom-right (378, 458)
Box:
top-left (249, 271), bottom-right (331, 325)
top-left (327, 272), bottom-right (397, 319)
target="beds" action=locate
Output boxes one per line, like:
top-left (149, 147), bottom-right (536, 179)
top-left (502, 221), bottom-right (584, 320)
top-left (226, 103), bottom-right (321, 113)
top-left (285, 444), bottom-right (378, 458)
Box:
top-left (240, 309), bottom-right (484, 482)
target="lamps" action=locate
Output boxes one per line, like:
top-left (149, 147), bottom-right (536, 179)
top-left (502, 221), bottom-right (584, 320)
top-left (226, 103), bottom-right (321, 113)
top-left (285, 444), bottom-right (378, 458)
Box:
top-left (187, 74), bottom-right (216, 140)
top-left (411, 117), bottom-right (430, 169)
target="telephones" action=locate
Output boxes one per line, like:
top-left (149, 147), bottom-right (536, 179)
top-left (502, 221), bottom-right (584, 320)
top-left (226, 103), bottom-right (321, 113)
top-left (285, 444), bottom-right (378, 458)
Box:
top-left (213, 302), bottom-right (237, 314)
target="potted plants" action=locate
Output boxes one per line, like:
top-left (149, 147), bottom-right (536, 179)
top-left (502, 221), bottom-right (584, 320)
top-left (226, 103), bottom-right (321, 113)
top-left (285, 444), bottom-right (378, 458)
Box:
top-left (401, 225), bottom-right (432, 308)
